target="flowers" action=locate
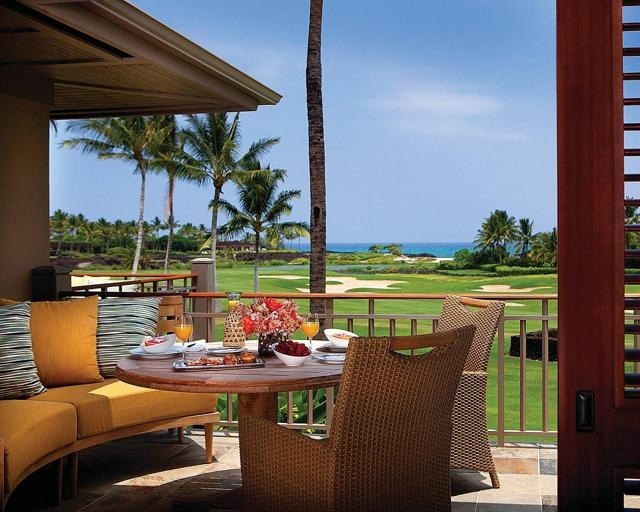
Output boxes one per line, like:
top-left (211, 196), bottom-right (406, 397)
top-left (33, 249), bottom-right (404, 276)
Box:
top-left (238, 297), bottom-right (304, 336)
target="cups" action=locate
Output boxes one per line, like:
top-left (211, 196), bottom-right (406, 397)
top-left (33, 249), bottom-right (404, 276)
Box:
top-left (227, 291), bottom-right (240, 305)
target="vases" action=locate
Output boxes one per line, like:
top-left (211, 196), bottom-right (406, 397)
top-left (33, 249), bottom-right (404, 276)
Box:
top-left (254, 332), bottom-right (289, 357)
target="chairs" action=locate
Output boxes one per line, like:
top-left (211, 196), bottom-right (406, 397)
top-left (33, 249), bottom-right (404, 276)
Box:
top-left (426, 291), bottom-right (508, 491)
top-left (232, 321), bottom-right (481, 512)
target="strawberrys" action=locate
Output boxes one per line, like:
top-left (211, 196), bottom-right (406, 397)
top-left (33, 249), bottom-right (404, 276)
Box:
top-left (275, 340), bottom-right (310, 357)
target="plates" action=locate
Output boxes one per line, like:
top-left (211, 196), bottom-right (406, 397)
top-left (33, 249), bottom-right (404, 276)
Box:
top-left (129, 347), bottom-right (191, 361)
top-left (172, 356), bottom-right (266, 370)
top-left (313, 354), bottom-right (345, 365)
top-left (205, 346), bottom-right (247, 357)
top-left (315, 346), bottom-right (348, 354)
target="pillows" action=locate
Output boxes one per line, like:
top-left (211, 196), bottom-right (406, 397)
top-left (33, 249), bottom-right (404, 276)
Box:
top-left (0, 294), bottom-right (50, 400)
top-left (0, 297), bottom-right (109, 388)
top-left (63, 294), bottom-right (171, 381)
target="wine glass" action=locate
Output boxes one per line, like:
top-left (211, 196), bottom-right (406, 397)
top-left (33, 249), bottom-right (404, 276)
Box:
top-left (174, 313), bottom-right (194, 360)
top-left (302, 314), bottom-right (320, 359)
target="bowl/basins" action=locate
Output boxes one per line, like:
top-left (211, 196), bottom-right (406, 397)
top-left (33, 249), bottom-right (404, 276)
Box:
top-left (141, 333), bottom-right (178, 352)
top-left (272, 342), bottom-right (312, 368)
top-left (323, 328), bottom-right (360, 348)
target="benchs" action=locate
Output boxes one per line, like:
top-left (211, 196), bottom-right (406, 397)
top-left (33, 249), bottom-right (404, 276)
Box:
top-left (0, 291), bottom-right (223, 512)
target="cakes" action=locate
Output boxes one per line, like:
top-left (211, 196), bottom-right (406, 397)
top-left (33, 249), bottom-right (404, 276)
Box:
top-left (239, 351), bottom-right (258, 363)
